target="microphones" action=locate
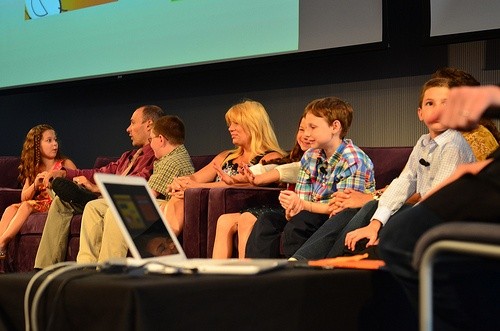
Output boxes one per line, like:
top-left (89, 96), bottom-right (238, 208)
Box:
top-left (419, 157), bottom-right (430, 167)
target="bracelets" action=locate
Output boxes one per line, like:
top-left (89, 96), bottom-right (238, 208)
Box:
top-left (372, 190), bottom-right (382, 200)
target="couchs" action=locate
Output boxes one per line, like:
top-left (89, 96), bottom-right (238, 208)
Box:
top-left (206, 145), bottom-right (414, 260)
top-left (0, 155), bottom-right (215, 271)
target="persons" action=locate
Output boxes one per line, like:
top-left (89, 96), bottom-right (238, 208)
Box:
top-left (292, 67), bottom-right (500, 259)
top-left (164, 100), bottom-right (289, 238)
top-left (0, 125), bottom-right (76, 259)
top-left (344, 78), bottom-right (478, 256)
top-left (141, 235), bottom-right (179, 256)
top-left (370, 85), bottom-right (500, 331)
top-left (76, 115), bottom-right (195, 270)
top-left (34, 105), bottom-right (167, 272)
top-left (244, 96), bottom-right (376, 260)
top-left (211, 114), bottom-right (310, 259)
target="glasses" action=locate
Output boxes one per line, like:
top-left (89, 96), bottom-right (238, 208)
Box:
top-left (148, 135), bottom-right (168, 142)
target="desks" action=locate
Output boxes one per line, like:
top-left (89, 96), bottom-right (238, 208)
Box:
top-left (0, 258), bottom-right (408, 331)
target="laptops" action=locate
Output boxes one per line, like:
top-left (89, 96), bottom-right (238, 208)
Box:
top-left (94, 173), bottom-right (287, 275)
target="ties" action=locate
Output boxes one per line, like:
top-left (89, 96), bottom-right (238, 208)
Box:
top-left (122, 149), bottom-right (143, 176)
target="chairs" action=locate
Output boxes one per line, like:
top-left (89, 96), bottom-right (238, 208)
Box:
top-left (410, 221), bottom-right (500, 331)
